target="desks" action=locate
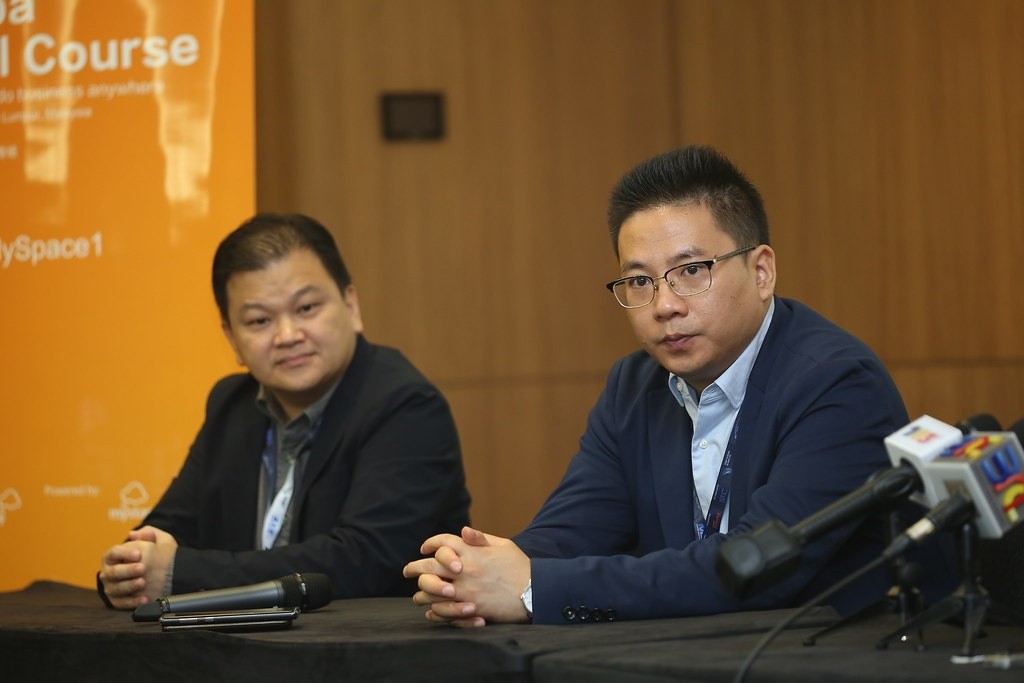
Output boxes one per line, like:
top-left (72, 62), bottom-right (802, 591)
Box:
top-left (0, 580), bottom-right (1024, 683)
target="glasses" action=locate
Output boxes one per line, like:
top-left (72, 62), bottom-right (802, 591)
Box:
top-left (607, 246), bottom-right (756, 309)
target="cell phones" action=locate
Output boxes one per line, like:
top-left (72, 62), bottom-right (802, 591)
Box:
top-left (159, 605), bottom-right (299, 633)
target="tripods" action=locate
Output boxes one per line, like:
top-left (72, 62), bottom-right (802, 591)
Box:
top-left (875, 519), bottom-right (1023, 657)
top-left (802, 502), bottom-right (987, 652)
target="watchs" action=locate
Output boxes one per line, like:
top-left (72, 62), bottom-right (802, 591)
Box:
top-left (519, 579), bottom-right (533, 622)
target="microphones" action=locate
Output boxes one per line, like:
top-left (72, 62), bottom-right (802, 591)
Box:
top-left (884, 416), bottom-right (1024, 562)
top-left (162, 572), bottom-right (332, 614)
top-left (720, 413), bottom-right (1002, 589)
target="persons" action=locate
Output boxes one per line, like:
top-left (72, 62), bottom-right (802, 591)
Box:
top-left (97, 212), bottom-right (472, 613)
top-left (402, 146), bottom-right (912, 630)
